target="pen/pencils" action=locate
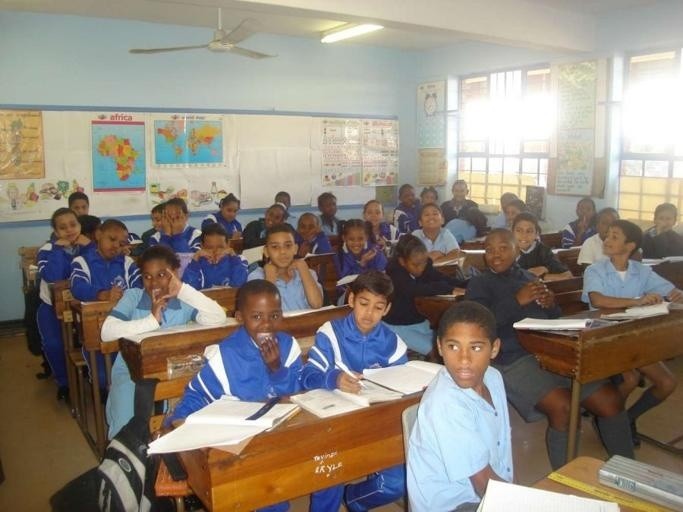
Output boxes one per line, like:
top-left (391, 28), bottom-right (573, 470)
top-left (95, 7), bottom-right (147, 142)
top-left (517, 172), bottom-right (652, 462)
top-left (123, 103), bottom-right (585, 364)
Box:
top-left (161, 307), bottom-right (168, 324)
top-left (334, 359), bottom-right (369, 390)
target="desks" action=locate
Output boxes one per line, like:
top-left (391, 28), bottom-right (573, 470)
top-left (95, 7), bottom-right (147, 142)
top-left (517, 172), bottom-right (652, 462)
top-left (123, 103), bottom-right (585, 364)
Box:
top-left (516, 298), bottom-right (683, 464)
top-left (169, 360), bottom-right (442, 507)
top-left (509, 457), bottom-right (683, 512)
top-left (21, 234), bottom-right (299, 321)
top-left (305, 221), bottom-right (680, 302)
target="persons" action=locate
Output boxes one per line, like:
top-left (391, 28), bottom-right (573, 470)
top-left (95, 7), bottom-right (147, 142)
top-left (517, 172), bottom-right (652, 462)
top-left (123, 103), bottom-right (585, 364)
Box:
top-left (142, 203), bottom-right (164, 244)
top-left (242, 203), bottom-right (294, 248)
top-left (577, 208), bottom-right (642, 273)
top-left (440, 180), bottom-right (478, 225)
top-left (295, 213), bottom-right (333, 259)
top-left (246, 224), bottom-right (324, 312)
top-left (36, 207), bottom-right (91, 401)
top-left (363, 200), bottom-right (407, 247)
top-left (303, 270), bottom-right (409, 512)
top-left (393, 184), bottom-right (425, 233)
top-left (504, 199), bottom-right (541, 244)
top-left (316, 192), bottom-right (344, 236)
top-left (50, 191), bottom-right (89, 243)
top-left (201, 193), bottom-right (246, 240)
top-left (415, 187), bottom-right (438, 211)
top-left (642, 203), bottom-right (683, 258)
top-left (407, 301), bottom-right (513, 512)
top-left (512, 210), bottom-right (573, 283)
top-left (580, 219), bottom-right (683, 459)
top-left (380, 234), bottom-right (470, 357)
top-left (164, 279), bottom-right (304, 512)
top-left (493, 192), bottom-right (518, 228)
top-left (411, 202), bottom-right (460, 260)
top-left (275, 191), bottom-right (299, 233)
top-left (101, 245), bottom-right (226, 440)
top-left (69, 219), bottom-right (145, 404)
top-left (146, 198), bottom-right (202, 253)
top-left (334, 219), bottom-right (387, 305)
top-left (443, 206), bottom-right (486, 246)
top-left (77, 215), bottom-right (143, 244)
top-left (181, 222), bottom-right (249, 290)
top-left (466, 229), bottom-right (636, 470)
top-left (561, 197), bottom-right (597, 248)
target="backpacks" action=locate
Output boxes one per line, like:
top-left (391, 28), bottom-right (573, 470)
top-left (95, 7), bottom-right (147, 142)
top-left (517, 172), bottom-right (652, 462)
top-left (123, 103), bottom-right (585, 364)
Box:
top-left (50, 398), bottom-right (187, 512)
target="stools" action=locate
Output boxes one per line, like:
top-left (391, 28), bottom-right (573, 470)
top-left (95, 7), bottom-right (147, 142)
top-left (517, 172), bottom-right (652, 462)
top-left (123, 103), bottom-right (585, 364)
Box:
top-left (38, 324), bottom-right (122, 458)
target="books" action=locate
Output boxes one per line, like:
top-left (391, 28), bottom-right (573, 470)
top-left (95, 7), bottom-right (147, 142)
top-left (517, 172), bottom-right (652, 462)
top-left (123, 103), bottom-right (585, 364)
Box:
top-left (290, 378), bottom-right (403, 419)
top-left (600, 296), bottom-right (683, 320)
top-left (362, 359), bottom-right (446, 396)
top-left (282, 304), bottom-right (348, 318)
top-left (476, 479), bottom-right (620, 512)
top-left (125, 316), bottom-right (246, 344)
top-left (146, 399), bottom-right (301, 456)
top-left (513, 317), bottom-right (620, 330)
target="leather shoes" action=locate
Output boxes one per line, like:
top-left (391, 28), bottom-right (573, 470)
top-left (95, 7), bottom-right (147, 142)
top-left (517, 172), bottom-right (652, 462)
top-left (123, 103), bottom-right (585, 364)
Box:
top-left (630, 422), bottom-right (640, 446)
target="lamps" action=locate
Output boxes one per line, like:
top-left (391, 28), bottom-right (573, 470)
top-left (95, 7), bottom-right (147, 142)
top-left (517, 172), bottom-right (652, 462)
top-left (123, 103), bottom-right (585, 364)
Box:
top-left (321, 20), bottom-right (385, 46)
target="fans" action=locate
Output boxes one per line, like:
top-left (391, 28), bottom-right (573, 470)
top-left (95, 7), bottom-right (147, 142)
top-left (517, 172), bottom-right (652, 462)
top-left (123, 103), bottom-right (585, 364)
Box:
top-left (129, 9), bottom-right (272, 61)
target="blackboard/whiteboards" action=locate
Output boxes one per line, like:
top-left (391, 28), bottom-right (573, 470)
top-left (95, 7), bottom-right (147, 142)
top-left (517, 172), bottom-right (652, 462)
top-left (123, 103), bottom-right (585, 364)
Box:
top-left (0, 103), bottom-right (401, 230)
